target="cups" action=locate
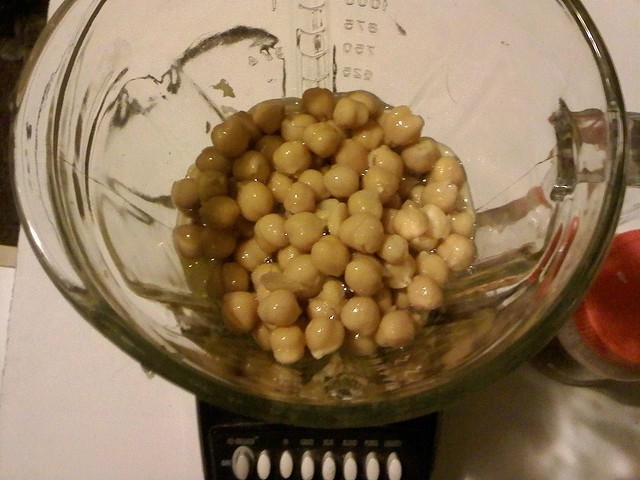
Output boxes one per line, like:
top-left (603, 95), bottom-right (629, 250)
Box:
top-left (2, 1), bottom-right (636, 434)
